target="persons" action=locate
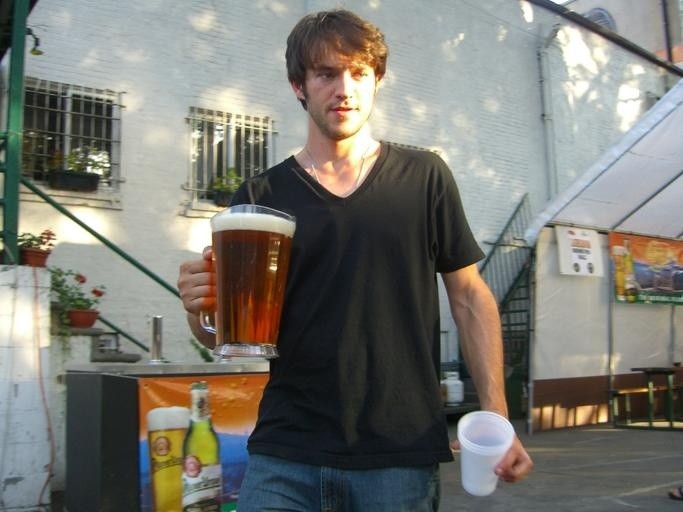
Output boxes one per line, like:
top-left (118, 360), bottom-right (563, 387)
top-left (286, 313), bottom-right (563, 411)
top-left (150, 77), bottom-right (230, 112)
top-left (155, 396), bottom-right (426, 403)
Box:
top-left (177, 7), bottom-right (534, 509)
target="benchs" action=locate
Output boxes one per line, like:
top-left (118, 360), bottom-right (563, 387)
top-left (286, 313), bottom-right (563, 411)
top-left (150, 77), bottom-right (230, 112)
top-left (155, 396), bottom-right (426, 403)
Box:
top-left (604, 382), bottom-right (682, 425)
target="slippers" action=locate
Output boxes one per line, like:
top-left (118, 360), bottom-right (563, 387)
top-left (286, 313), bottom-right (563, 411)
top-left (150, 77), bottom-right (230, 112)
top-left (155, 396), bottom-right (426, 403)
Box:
top-left (668, 486), bottom-right (683, 500)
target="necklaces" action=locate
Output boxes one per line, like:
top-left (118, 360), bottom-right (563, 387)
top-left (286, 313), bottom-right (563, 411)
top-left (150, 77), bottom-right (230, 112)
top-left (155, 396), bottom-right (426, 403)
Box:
top-left (303, 141), bottom-right (367, 198)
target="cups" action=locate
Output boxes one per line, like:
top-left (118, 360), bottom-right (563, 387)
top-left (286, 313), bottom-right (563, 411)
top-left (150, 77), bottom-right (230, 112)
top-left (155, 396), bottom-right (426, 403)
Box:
top-left (613, 255), bottom-right (628, 301)
top-left (456, 410), bottom-right (515, 498)
top-left (200, 203), bottom-right (297, 358)
top-left (149, 428), bottom-right (188, 512)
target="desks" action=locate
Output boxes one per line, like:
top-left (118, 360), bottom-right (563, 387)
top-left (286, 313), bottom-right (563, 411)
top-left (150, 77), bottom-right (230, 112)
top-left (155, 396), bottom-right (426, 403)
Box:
top-left (631, 367), bottom-right (681, 428)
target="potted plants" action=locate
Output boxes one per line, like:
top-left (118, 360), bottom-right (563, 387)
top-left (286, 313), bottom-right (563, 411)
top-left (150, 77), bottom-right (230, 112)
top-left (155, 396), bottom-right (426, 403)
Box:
top-left (44, 146), bottom-right (112, 192)
top-left (210, 166), bottom-right (237, 206)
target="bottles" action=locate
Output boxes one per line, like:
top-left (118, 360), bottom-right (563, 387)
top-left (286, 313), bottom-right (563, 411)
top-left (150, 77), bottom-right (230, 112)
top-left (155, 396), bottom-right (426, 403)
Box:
top-left (180, 380), bottom-right (224, 512)
top-left (623, 238), bottom-right (638, 302)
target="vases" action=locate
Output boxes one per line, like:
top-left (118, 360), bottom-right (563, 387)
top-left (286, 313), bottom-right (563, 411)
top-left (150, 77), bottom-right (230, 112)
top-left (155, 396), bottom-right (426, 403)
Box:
top-left (66, 309), bottom-right (99, 328)
top-left (20, 248), bottom-right (50, 267)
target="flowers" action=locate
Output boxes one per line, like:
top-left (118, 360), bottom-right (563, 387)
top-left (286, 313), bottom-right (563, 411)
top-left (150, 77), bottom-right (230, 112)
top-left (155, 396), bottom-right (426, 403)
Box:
top-left (51, 269), bottom-right (106, 309)
top-left (16, 228), bottom-right (55, 247)
